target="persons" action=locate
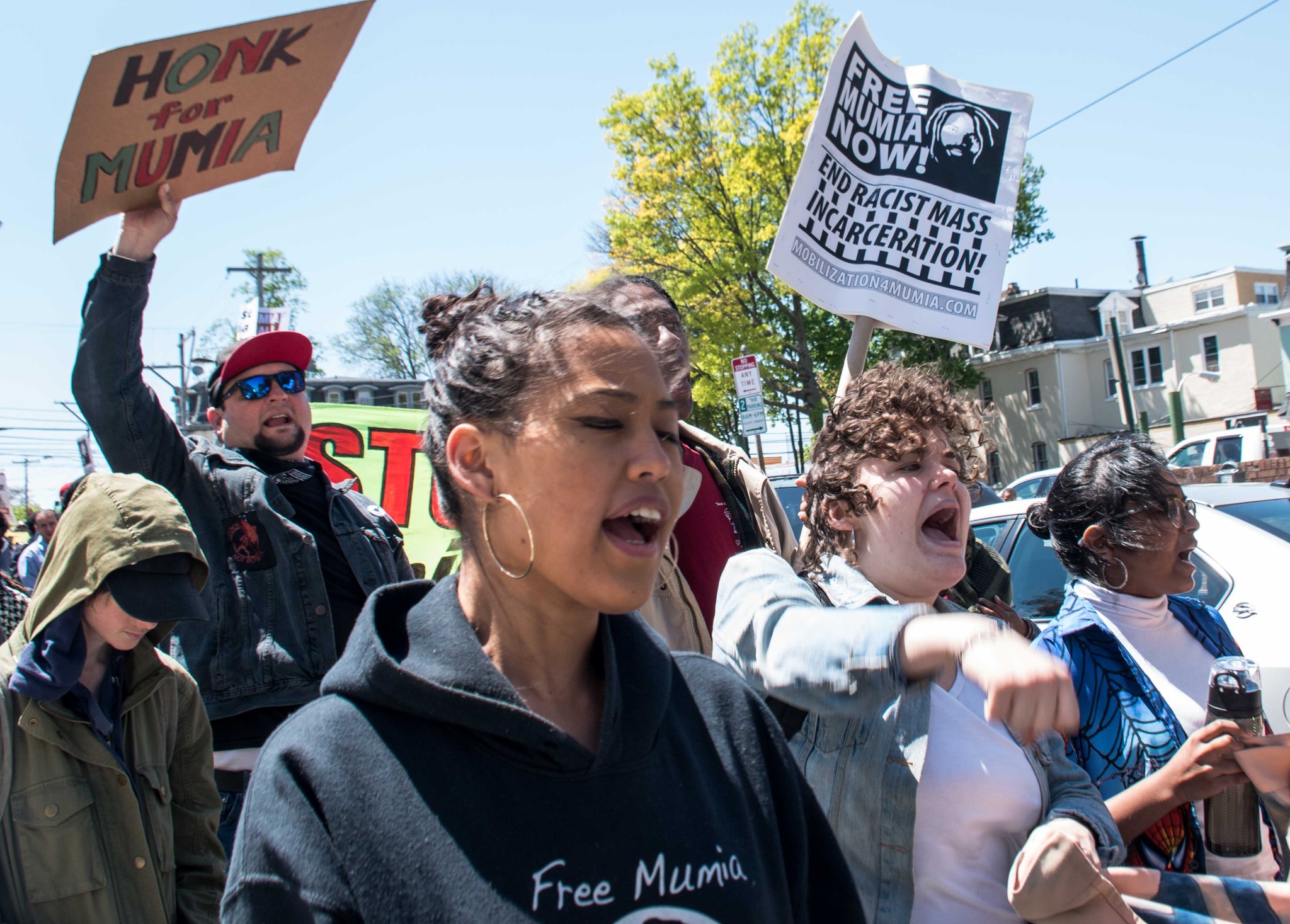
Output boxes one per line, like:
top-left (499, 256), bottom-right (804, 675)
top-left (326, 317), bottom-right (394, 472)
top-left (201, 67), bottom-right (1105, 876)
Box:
top-left (938, 525), bottom-right (1041, 642)
top-left (71, 182), bottom-right (416, 875)
top-left (586, 274), bottom-right (809, 659)
top-left (0, 473), bottom-right (73, 646)
top-left (0, 472), bottom-right (229, 924)
top-left (711, 358), bottom-right (1127, 924)
top-left (1001, 488), bottom-right (1017, 502)
top-left (1027, 431), bottom-right (1290, 883)
top-left (219, 277), bottom-right (868, 924)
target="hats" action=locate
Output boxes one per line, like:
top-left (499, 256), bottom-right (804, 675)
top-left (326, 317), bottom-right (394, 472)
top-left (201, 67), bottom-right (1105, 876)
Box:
top-left (207, 331), bottom-right (313, 406)
top-left (104, 552), bottom-right (210, 623)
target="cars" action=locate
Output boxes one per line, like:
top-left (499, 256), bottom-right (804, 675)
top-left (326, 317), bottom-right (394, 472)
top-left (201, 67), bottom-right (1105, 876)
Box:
top-left (967, 498), bottom-right (1289, 737)
top-left (995, 467), bottom-right (1063, 498)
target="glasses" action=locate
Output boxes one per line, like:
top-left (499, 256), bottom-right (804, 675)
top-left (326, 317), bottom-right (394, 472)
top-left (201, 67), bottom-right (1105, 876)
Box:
top-left (212, 370), bottom-right (306, 404)
top-left (1113, 500), bottom-right (1196, 530)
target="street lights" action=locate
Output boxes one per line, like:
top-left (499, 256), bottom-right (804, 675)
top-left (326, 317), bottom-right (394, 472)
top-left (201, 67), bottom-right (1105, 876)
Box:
top-left (1164, 367), bottom-right (1220, 466)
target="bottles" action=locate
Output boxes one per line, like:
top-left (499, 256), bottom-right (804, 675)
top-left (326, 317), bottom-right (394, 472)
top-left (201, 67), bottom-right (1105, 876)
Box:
top-left (1204, 655), bottom-right (1263, 859)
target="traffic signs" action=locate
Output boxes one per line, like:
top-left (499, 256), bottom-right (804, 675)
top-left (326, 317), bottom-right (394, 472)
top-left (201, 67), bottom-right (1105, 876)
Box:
top-left (738, 394), bottom-right (767, 437)
top-left (731, 355), bottom-right (762, 398)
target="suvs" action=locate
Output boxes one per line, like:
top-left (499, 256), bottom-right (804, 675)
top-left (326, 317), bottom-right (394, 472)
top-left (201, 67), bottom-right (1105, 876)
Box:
top-left (768, 473), bottom-right (1009, 545)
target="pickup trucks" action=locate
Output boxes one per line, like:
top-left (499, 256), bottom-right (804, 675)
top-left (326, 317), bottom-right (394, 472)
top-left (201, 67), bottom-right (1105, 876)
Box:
top-left (1165, 425), bottom-right (1266, 469)
